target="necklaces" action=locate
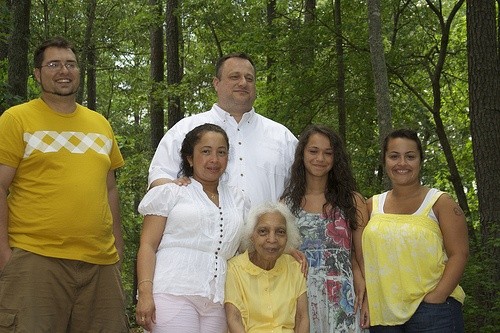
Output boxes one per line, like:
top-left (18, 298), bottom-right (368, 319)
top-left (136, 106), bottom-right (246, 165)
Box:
top-left (204, 188), bottom-right (217, 201)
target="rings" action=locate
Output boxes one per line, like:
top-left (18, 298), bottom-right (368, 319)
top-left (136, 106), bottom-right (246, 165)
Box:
top-left (140, 316), bottom-right (146, 322)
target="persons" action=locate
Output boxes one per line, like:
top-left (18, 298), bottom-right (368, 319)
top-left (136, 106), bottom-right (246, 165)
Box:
top-left (0, 36), bottom-right (131, 333)
top-left (279, 125), bottom-right (370, 333)
top-left (146, 52), bottom-right (299, 204)
top-left (224, 201), bottom-right (310, 333)
top-left (352, 128), bottom-right (470, 333)
top-left (136, 123), bottom-right (308, 333)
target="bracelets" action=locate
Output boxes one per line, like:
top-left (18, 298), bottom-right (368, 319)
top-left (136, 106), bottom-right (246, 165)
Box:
top-left (138, 279), bottom-right (154, 287)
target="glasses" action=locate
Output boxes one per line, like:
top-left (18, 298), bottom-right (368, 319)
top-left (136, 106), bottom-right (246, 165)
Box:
top-left (41, 61), bottom-right (78, 70)
